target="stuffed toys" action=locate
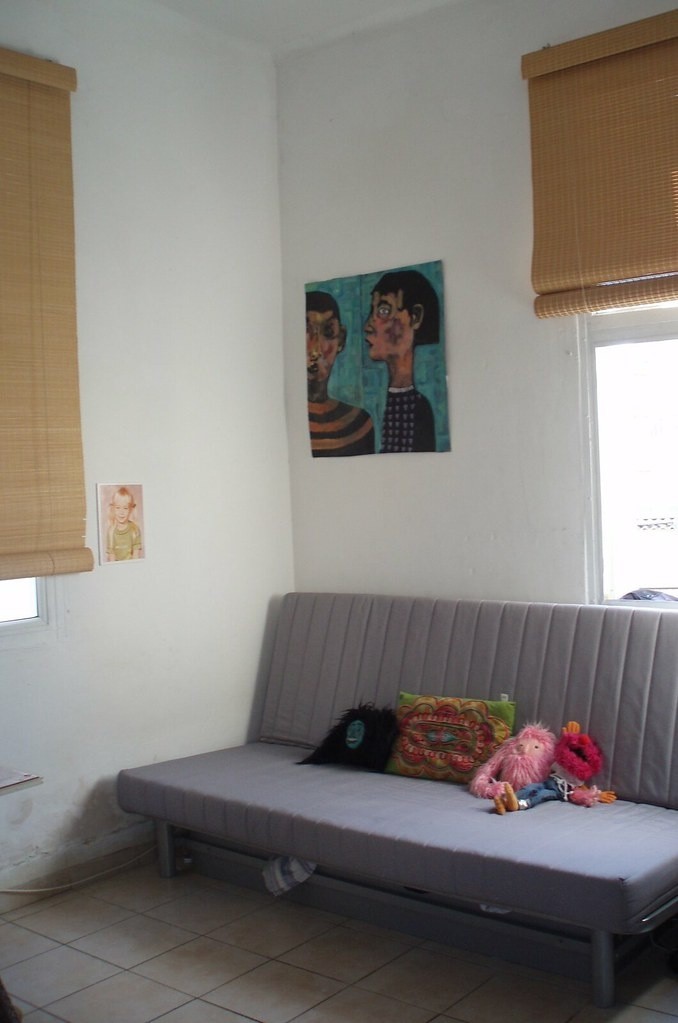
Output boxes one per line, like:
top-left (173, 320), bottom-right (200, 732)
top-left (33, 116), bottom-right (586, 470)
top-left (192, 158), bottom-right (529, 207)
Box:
top-left (469, 722), bottom-right (617, 815)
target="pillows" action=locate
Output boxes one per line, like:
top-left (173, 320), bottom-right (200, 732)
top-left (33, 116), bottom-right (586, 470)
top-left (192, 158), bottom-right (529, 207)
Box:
top-left (382, 689), bottom-right (515, 785)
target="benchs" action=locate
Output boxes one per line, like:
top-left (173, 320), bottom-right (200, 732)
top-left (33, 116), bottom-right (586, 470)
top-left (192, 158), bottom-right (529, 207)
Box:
top-left (114, 590), bottom-right (678, 1009)
